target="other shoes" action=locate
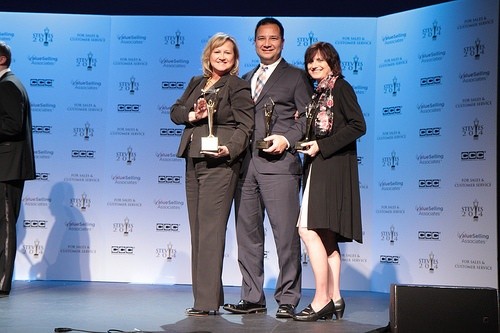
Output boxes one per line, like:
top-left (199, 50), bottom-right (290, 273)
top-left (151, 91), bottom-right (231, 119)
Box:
top-left (185, 307), bottom-right (219, 317)
top-left (0, 290), bottom-right (10, 297)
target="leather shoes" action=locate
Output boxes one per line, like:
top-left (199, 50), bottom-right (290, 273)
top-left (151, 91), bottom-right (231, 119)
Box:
top-left (276, 303), bottom-right (296, 317)
top-left (223, 299), bottom-right (267, 313)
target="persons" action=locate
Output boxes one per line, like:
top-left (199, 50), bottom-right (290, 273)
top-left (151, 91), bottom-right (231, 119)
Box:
top-left (222, 17), bottom-right (314, 318)
top-left (294, 41), bottom-right (367, 322)
top-left (170, 32), bottom-right (255, 318)
top-left (0, 39), bottom-right (37, 299)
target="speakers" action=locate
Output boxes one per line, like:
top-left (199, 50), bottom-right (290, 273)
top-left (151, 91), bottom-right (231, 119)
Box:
top-left (388, 284), bottom-right (500, 333)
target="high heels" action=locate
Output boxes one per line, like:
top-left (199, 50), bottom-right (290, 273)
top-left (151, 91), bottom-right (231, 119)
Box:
top-left (293, 297), bottom-right (335, 321)
top-left (320, 297), bottom-right (345, 320)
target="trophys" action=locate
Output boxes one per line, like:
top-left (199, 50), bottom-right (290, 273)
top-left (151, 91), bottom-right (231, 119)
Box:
top-left (200, 87), bottom-right (223, 152)
top-left (295, 102), bottom-right (320, 150)
top-left (255, 96), bottom-right (275, 149)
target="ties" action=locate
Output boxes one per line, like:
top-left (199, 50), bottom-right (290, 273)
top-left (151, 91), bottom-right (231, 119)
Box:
top-left (254, 65), bottom-right (269, 103)
